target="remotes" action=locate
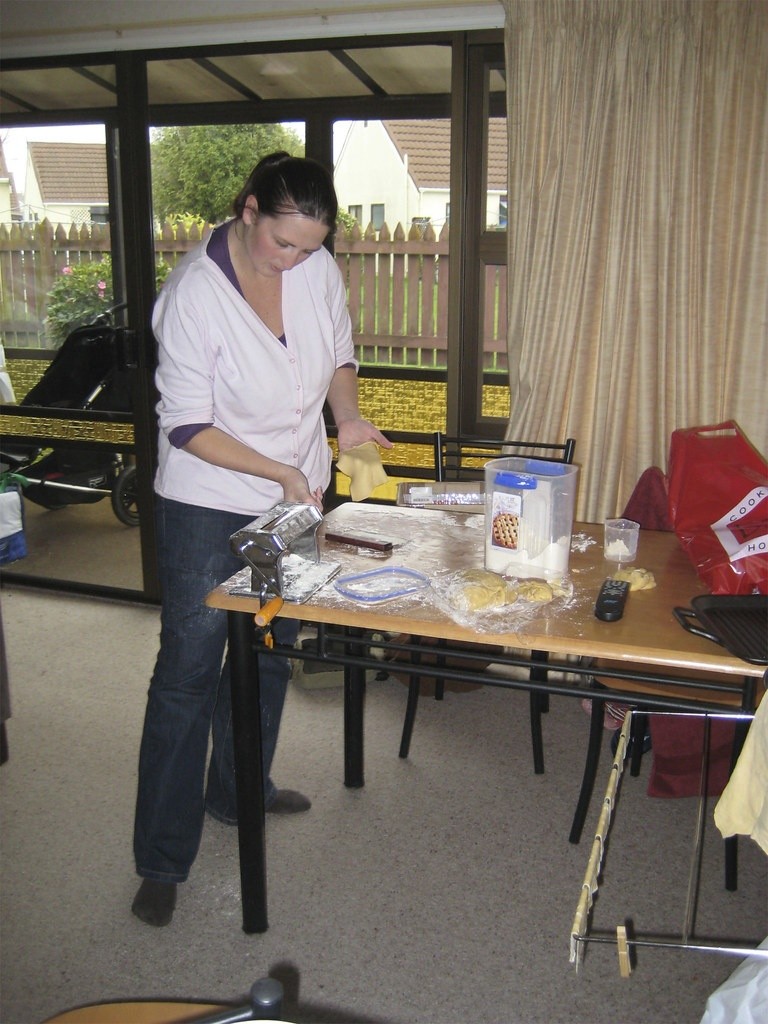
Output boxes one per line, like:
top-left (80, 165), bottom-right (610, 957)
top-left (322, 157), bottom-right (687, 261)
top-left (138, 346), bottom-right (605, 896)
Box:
top-left (595, 579), bottom-right (631, 623)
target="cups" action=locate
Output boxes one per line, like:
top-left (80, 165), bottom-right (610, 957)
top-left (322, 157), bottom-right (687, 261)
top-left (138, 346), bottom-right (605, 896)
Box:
top-left (604, 518), bottom-right (640, 563)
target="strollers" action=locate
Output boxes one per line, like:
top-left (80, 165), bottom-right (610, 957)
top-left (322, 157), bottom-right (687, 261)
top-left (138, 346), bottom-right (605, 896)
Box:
top-left (1, 303), bottom-right (143, 529)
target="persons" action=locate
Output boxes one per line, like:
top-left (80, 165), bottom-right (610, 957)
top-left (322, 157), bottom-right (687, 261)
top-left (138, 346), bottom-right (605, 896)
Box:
top-left (128, 151), bottom-right (394, 927)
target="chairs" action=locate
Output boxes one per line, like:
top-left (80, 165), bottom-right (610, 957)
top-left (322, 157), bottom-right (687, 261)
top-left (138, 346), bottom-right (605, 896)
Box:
top-left (399, 432), bottom-right (577, 775)
top-left (569, 465), bottom-right (759, 891)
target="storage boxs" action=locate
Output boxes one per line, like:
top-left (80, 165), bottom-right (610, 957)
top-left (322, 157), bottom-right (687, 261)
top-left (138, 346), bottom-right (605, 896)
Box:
top-left (483, 451), bottom-right (584, 579)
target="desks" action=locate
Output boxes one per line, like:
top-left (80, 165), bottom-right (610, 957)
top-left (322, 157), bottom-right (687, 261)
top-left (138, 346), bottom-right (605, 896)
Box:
top-left (203, 503), bottom-right (767, 933)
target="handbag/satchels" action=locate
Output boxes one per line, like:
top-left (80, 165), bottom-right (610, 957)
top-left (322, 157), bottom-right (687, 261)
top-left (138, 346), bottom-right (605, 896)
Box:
top-left (669, 420), bottom-right (768, 595)
